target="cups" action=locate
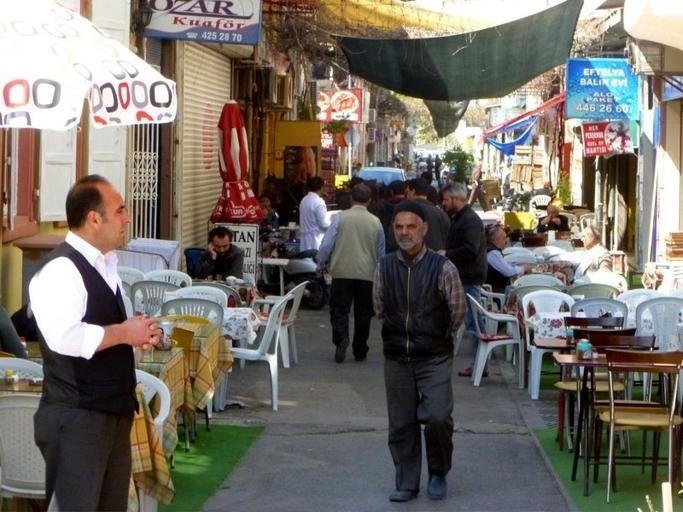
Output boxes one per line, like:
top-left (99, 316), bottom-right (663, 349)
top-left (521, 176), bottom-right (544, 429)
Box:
top-left (226, 276), bottom-right (237, 286)
top-left (207, 275), bottom-right (213, 283)
top-left (216, 275), bottom-right (222, 283)
top-left (288, 222), bottom-right (296, 228)
top-left (535, 256), bottom-right (544, 262)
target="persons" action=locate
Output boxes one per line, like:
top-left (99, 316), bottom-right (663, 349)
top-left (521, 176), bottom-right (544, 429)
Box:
top-left (25, 176), bottom-right (164, 511)
top-left (372, 202), bottom-right (468, 502)
top-left (196, 226), bottom-right (248, 308)
top-left (258, 155), bottom-right (613, 377)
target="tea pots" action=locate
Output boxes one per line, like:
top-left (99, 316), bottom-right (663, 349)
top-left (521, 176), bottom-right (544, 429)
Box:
top-left (151, 320), bottom-right (177, 351)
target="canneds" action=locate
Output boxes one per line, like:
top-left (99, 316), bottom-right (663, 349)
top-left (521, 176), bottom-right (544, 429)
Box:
top-left (154, 321), bottom-right (173, 351)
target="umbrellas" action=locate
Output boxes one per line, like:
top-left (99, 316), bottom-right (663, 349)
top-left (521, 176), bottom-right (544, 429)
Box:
top-left (0, 0), bottom-right (178, 131)
top-left (210, 100), bottom-right (265, 225)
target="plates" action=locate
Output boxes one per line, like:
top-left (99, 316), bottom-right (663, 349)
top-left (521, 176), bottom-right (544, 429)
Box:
top-left (331, 91), bottom-right (361, 113)
top-left (316, 91), bottom-right (330, 112)
top-left (235, 282), bottom-right (248, 286)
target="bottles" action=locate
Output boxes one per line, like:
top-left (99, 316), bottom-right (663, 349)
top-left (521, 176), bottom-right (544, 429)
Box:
top-left (20, 337), bottom-right (29, 360)
top-left (576, 337), bottom-right (592, 361)
top-left (565, 328), bottom-right (574, 346)
top-left (4, 370), bottom-right (19, 384)
top-left (592, 348), bottom-right (598, 360)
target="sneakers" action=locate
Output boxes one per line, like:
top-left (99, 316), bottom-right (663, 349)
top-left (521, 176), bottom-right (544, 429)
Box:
top-left (333, 336), bottom-right (349, 364)
top-left (457, 364), bottom-right (489, 378)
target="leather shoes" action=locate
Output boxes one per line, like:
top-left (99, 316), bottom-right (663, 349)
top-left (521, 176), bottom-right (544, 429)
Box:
top-left (387, 486), bottom-right (419, 503)
top-left (425, 473), bottom-right (448, 499)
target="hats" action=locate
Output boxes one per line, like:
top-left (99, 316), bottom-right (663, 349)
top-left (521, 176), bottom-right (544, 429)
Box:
top-left (391, 198), bottom-right (426, 222)
